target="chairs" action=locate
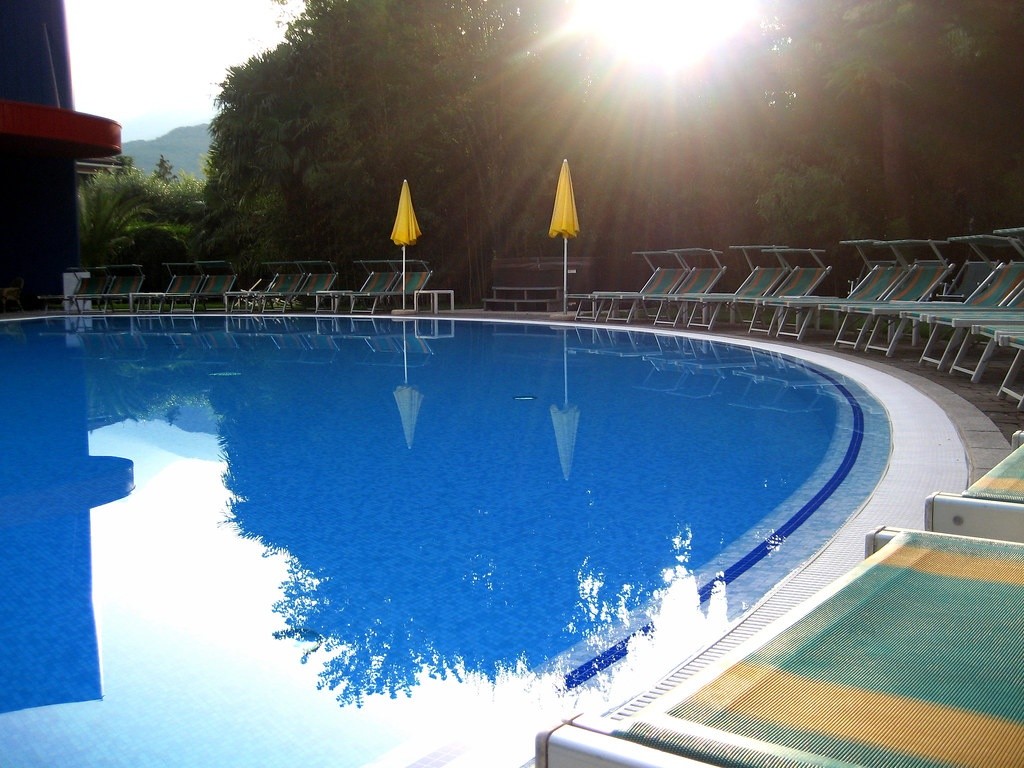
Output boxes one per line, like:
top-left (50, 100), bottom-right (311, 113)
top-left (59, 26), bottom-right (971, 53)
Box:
top-left (535, 226), bottom-right (1024, 768)
top-left (37, 258), bottom-right (433, 315)
top-left (45, 317), bottom-right (435, 358)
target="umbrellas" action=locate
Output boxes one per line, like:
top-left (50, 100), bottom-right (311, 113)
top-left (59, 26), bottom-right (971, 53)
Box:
top-left (389, 180), bottom-right (422, 309)
top-left (551, 330), bottom-right (581, 478)
top-left (393, 321), bottom-right (423, 448)
top-left (547, 160), bottom-right (581, 313)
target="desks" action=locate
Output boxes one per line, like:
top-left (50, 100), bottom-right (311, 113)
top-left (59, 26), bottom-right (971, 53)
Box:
top-left (414, 319), bottom-right (454, 339)
top-left (226, 289), bottom-right (261, 312)
top-left (413, 288), bottom-right (455, 313)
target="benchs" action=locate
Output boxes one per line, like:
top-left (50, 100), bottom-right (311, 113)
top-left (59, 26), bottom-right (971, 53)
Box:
top-left (482, 286), bottom-right (567, 313)
